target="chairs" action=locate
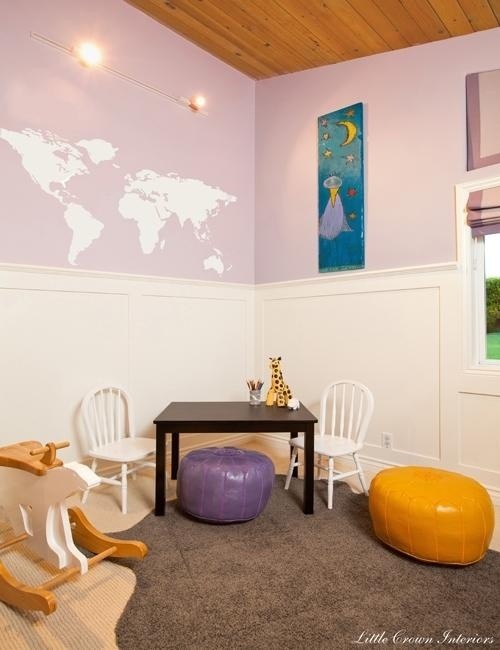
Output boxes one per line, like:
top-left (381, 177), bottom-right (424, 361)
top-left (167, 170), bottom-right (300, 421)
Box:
top-left (284, 380), bottom-right (370, 511)
top-left (80, 384), bottom-right (167, 517)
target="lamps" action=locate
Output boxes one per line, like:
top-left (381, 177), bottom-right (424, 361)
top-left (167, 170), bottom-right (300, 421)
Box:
top-left (30, 28), bottom-right (208, 121)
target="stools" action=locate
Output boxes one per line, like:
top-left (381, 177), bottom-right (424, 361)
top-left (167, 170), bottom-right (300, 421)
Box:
top-left (175, 443), bottom-right (276, 523)
top-left (368, 464), bottom-right (494, 567)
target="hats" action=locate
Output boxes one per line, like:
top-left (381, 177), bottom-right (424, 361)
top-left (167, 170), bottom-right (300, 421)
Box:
top-left (323, 176), bottom-right (342, 188)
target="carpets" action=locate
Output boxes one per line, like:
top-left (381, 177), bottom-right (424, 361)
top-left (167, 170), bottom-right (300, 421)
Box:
top-left (92, 474), bottom-right (500, 650)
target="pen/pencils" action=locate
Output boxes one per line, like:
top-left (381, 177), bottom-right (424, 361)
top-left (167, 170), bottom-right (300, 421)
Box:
top-left (247, 378), bottom-right (264, 390)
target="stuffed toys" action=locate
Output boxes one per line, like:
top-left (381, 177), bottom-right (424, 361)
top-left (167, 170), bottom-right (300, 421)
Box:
top-left (266, 356), bottom-right (292, 407)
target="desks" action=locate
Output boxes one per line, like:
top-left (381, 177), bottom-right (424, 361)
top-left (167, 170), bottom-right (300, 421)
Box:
top-left (150, 400), bottom-right (318, 515)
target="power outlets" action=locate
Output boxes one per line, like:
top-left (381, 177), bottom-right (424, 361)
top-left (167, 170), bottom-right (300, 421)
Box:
top-left (380, 433), bottom-right (391, 450)
